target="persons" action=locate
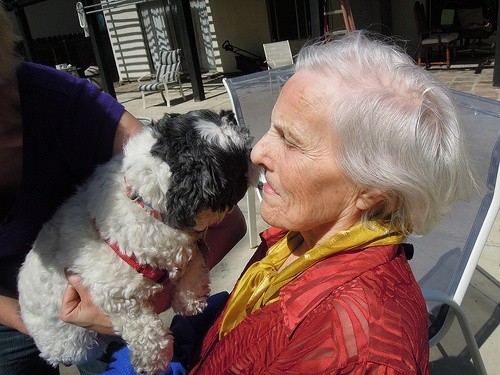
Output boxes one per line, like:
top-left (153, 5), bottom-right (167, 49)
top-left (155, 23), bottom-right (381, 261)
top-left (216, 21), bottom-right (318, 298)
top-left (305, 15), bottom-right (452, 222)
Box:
top-left (0, 7), bottom-right (248, 375)
top-left (187, 29), bottom-right (486, 375)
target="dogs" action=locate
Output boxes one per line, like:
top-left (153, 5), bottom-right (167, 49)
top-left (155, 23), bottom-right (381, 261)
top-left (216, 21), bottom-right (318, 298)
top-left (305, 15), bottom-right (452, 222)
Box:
top-left (17, 109), bottom-right (252, 375)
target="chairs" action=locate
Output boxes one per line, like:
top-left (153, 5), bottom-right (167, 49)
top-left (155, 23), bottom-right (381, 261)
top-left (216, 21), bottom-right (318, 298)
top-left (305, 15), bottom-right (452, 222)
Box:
top-left (413, 0), bottom-right (499, 69)
top-left (222, 63), bottom-right (297, 249)
top-left (263, 40), bottom-right (300, 94)
top-left (402, 88), bottom-right (500, 375)
top-left (138, 49), bottom-right (187, 109)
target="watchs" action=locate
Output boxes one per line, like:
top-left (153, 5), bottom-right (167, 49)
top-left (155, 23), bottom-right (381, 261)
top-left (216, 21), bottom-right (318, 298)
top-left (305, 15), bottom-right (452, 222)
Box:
top-left (196, 239), bottom-right (210, 258)
